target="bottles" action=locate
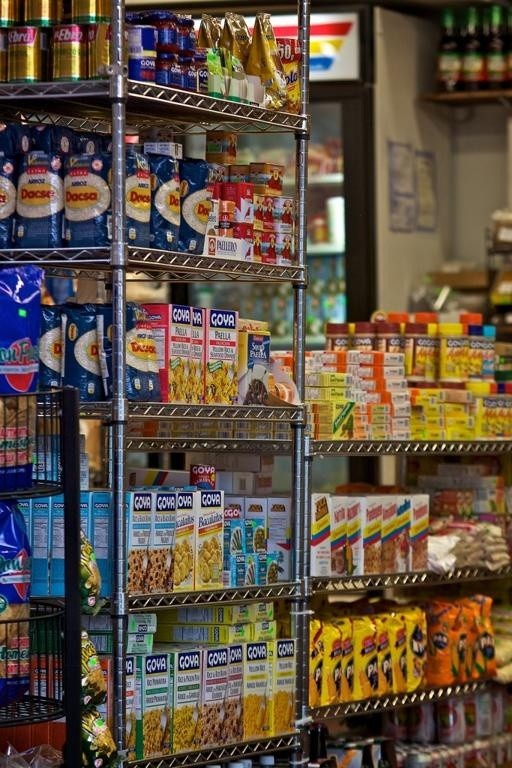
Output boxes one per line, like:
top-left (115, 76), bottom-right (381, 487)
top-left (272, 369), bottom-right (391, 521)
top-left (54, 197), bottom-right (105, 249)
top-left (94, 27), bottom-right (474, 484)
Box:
top-left (188, 253), bottom-right (348, 340)
top-left (433, 6), bottom-right (512, 91)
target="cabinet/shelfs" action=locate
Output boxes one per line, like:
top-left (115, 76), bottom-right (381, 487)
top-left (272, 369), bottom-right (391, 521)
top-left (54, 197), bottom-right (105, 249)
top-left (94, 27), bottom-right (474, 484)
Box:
top-left (0, 0), bottom-right (512, 768)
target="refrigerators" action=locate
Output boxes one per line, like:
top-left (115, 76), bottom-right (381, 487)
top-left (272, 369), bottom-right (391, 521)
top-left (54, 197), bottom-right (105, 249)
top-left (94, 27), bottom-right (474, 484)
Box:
top-left (172, 2), bottom-right (457, 351)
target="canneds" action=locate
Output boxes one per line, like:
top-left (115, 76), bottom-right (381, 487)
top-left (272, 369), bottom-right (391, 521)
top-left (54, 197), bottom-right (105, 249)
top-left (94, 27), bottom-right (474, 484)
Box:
top-left (0, 0), bottom-right (208, 94)
top-left (327, 689), bottom-right (512, 768)
top-left (205, 129), bottom-right (300, 267)
